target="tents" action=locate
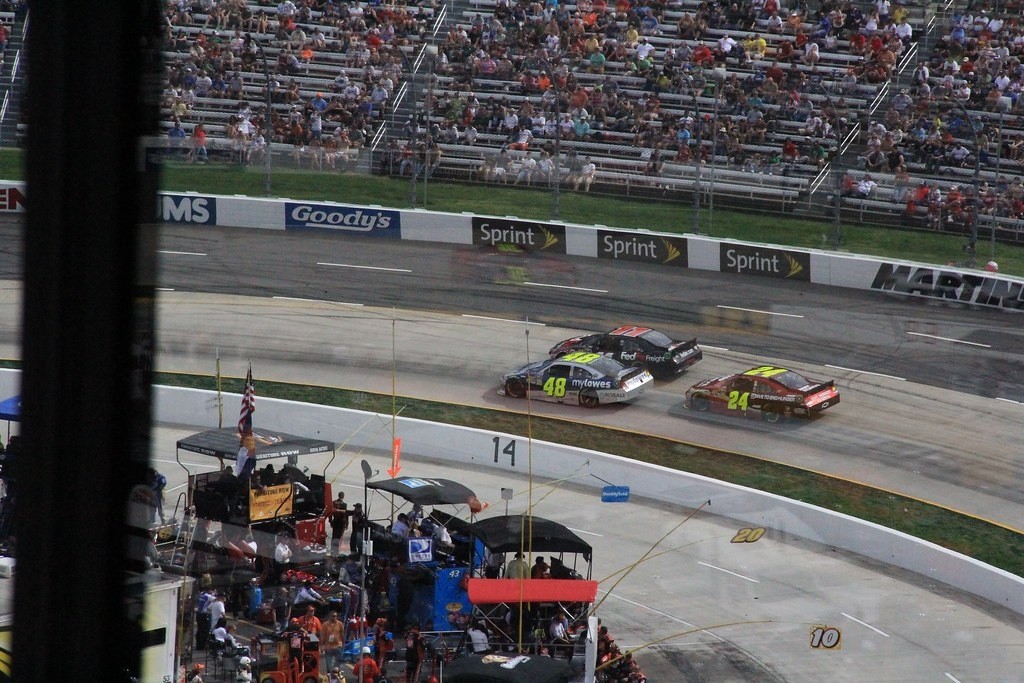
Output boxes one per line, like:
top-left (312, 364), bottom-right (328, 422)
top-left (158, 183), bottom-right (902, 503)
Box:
top-left (177, 428), bottom-right (337, 480)
top-left (365, 476), bottom-right (478, 546)
top-left (471, 514), bottom-right (594, 612)
top-left (440, 651), bottom-right (572, 683)
top-left (0, 395), bottom-right (21, 442)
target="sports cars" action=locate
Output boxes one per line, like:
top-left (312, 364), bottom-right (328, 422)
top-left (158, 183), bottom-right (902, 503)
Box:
top-left (546, 326), bottom-right (702, 380)
top-left (685, 365), bottom-right (841, 423)
top-left (501, 349), bottom-right (654, 409)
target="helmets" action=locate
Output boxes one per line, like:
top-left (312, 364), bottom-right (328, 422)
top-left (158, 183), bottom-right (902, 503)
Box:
top-left (240, 656), bottom-right (251, 665)
top-left (363, 646), bottom-right (370, 654)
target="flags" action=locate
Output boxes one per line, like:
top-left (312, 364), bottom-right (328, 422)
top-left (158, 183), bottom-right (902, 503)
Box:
top-left (238, 365), bottom-right (255, 479)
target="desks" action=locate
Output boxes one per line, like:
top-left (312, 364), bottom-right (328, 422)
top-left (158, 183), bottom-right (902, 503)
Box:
top-left (436, 521), bottom-right (475, 543)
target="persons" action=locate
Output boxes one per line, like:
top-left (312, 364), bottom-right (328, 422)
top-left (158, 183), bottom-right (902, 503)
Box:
top-left (0, 436), bottom-right (168, 570)
top-left (0, 0), bottom-right (1024, 225)
top-left (197, 465), bottom-right (647, 683)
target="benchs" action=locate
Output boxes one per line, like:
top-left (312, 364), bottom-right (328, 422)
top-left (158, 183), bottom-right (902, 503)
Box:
top-left (160, 0), bottom-right (1024, 245)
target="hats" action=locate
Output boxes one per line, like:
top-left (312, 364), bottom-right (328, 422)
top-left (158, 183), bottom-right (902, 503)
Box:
top-left (353, 503), bottom-right (362, 508)
top-left (310, 608), bottom-right (314, 611)
top-left (291, 618), bottom-right (299, 623)
top-left (195, 664), bottom-right (204, 669)
top-left (377, 618), bottom-right (387, 623)
top-left (218, 591), bottom-right (228, 597)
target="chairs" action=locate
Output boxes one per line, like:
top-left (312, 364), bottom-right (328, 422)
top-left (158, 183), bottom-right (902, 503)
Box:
top-left (205, 634), bottom-right (248, 682)
top-left (432, 538), bottom-right (454, 567)
top-left (541, 619), bottom-right (573, 660)
